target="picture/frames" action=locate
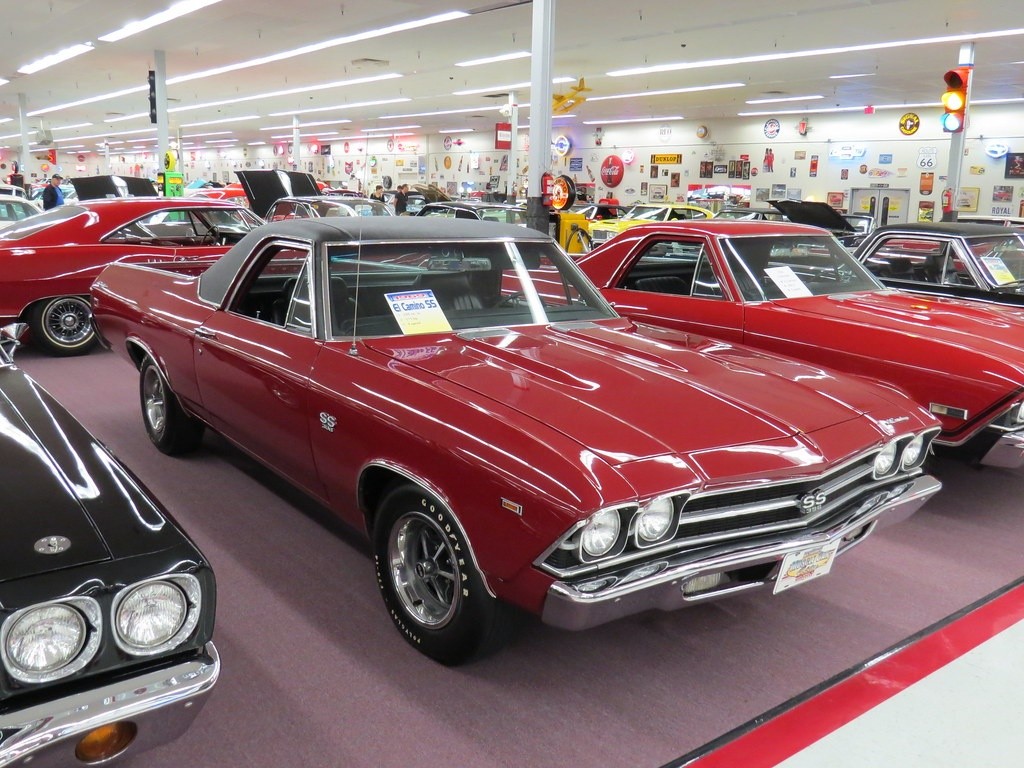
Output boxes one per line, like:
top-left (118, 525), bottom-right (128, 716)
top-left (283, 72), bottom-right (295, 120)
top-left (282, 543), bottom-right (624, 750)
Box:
top-left (1004, 153), bottom-right (1024, 178)
top-left (756, 183), bottom-right (801, 202)
top-left (700, 160), bottom-right (750, 180)
top-left (827, 192), bottom-right (844, 208)
top-left (641, 166), bottom-right (680, 203)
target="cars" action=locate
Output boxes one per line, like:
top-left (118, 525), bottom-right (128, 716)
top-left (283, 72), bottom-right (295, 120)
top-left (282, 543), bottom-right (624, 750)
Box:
top-left (89, 216), bottom-right (943, 667)
top-left (0, 170), bottom-right (527, 357)
top-left (0, 323), bottom-right (220, 768)
top-left (501, 200), bottom-right (1024, 470)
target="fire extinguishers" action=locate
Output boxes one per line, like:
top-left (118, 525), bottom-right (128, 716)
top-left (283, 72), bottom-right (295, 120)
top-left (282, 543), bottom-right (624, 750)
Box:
top-left (541, 170), bottom-right (556, 205)
top-left (941, 188), bottom-right (952, 213)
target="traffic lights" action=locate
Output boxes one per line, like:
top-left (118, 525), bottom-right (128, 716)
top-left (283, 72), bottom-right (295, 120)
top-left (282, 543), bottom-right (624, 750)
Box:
top-left (941, 67), bottom-right (968, 133)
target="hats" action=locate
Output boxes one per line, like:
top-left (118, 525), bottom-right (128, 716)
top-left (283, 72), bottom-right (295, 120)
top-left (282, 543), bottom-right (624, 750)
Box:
top-left (52, 174), bottom-right (63, 179)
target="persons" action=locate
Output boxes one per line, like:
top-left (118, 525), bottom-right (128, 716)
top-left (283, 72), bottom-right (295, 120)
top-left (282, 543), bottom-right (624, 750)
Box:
top-left (370, 185), bottom-right (385, 216)
top-left (394, 183), bottom-right (409, 216)
top-left (42, 174), bottom-right (64, 211)
top-left (208, 180), bottom-right (226, 188)
top-left (397, 185), bottom-right (401, 191)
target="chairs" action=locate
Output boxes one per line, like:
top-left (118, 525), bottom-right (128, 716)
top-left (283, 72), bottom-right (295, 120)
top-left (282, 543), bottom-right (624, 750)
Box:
top-left (633, 276), bottom-right (691, 295)
top-left (411, 269), bottom-right (485, 312)
top-left (730, 259), bottom-right (769, 301)
top-left (922, 255), bottom-right (961, 284)
top-left (271, 273), bottom-right (362, 326)
top-left (887, 258), bottom-right (916, 281)
